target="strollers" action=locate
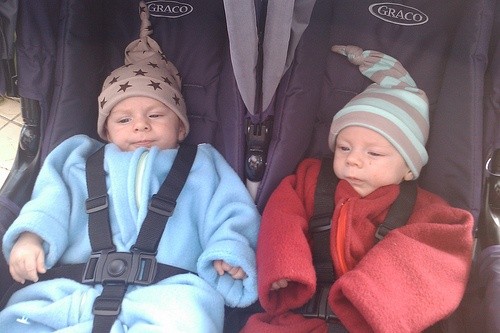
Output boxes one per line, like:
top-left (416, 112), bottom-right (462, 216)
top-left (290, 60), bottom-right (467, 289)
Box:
top-left (233, 0), bottom-right (500, 333)
top-left (1, 0), bottom-right (259, 333)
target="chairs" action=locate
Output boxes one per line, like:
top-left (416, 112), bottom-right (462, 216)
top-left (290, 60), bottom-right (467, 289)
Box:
top-left (0, 1), bottom-right (260, 333)
top-left (253, 1), bottom-right (500, 332)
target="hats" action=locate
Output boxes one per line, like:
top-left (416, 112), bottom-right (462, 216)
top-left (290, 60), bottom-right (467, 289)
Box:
top-left (326, 43), bottom-right (430, 178)
top-left (96, 4), bottom-right (191, 140)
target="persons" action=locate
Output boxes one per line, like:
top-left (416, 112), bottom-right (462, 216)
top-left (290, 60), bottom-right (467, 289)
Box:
top-left (236, 44), bottom-right (475, 333)
top-left (0, 0), bottom-right (500, 331)
top-left (0, 1), bottom-right (262, 333)
